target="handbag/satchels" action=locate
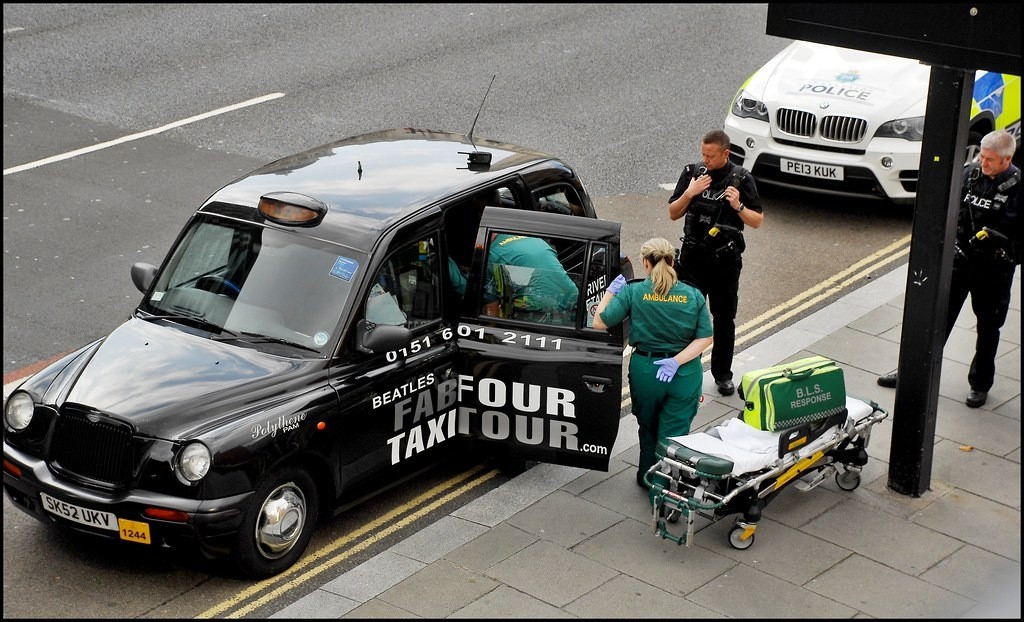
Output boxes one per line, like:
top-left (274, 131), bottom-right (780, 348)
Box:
top-left (737, 355), bottom-right (846, 432)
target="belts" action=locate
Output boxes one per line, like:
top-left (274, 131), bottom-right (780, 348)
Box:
top-left (635, 348), bottom-right (680, 357)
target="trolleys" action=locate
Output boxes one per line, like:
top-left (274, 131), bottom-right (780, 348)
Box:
top-left (642, 391), bottom-right (889, 549)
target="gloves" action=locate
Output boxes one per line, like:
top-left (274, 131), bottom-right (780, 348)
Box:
top-left (605, 274), bottom-right (628, 296)
top-left (652, 358), bottom-right (680, 383)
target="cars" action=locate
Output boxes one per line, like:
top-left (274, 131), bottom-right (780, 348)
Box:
top-left (0, 126), bottom-right (621, 575)
top-left (722, 35), bottom-right (1022, 206)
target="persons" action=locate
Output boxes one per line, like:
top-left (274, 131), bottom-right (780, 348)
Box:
top-left (444, 224), bottom-right (582, 327)
top-left (878, 129), bottom-right (1024, 410)
top-left (593, 237), bottom-right (715, 494)
top-left (668, 128), bottom-right (765, 398)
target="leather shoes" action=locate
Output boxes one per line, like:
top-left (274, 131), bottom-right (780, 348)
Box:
top-left (965, 388), bottom-right (988, 409)
top-left (714, 379), bottom-right (735, 397)
top-left (877, 372), bottom-right (897, 388)
